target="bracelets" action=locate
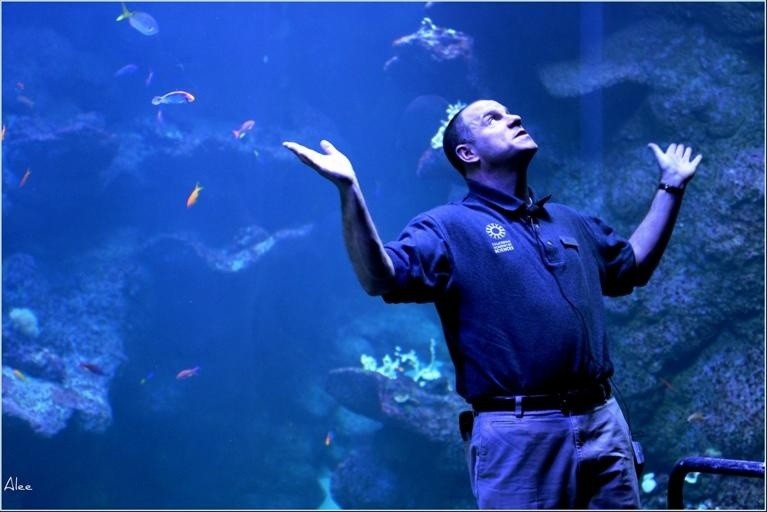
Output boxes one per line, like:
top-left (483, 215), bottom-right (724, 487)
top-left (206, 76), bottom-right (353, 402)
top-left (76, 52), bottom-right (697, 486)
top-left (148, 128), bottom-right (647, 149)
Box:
top-left (655, 181), bottom-right (683, 198)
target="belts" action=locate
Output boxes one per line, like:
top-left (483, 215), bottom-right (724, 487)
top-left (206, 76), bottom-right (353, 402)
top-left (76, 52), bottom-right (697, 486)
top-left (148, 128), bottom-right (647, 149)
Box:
top-left (473, 377), bottom-right (612, 416)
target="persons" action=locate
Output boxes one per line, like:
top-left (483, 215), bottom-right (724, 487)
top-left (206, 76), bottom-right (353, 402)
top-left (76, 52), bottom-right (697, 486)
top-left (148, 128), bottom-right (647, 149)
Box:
top-left (280, 97), bottom-right (704, 508)
